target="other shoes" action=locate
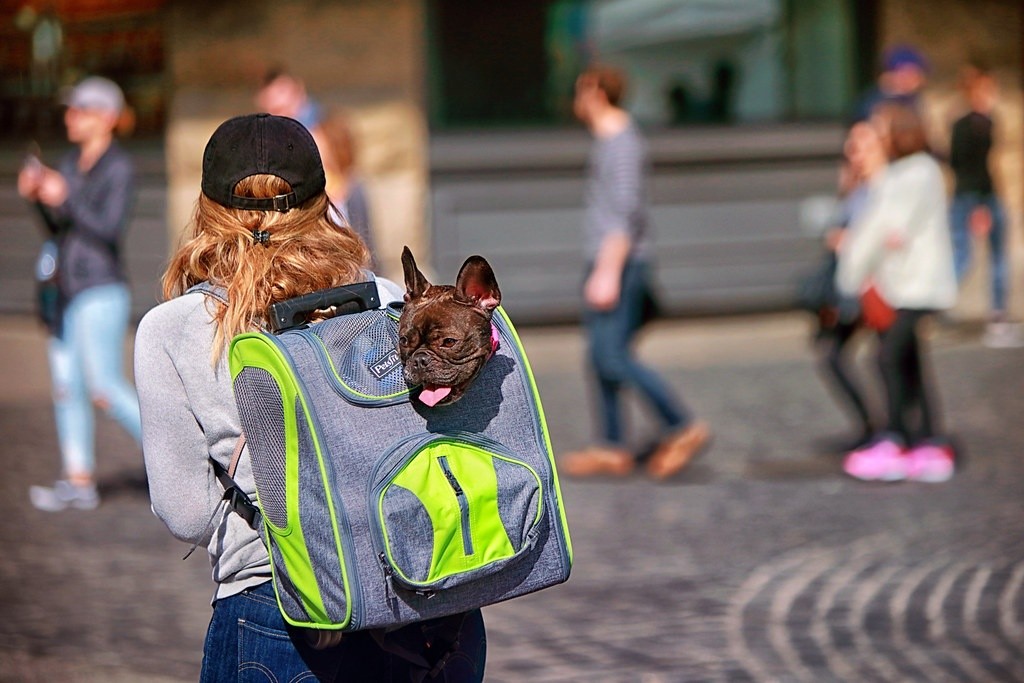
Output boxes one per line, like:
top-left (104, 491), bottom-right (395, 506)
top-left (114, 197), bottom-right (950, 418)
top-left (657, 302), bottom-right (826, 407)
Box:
top-left (557, 445), bottom-right (634, 481)
top-left (841, 439), bottom-right (954, 484)
top-left (29, 479), bottom-right (100, 512)
top-left (646, 421), bottom-right (714, 481)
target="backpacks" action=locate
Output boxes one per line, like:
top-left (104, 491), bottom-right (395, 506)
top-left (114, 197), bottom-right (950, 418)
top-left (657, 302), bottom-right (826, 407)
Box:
top-left (181, 270), bottom-right (575, 631)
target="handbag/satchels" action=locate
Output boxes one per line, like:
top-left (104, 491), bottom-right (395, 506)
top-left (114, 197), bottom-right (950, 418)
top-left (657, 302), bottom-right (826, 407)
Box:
top-left (32, 229), bottom-right (67, 340)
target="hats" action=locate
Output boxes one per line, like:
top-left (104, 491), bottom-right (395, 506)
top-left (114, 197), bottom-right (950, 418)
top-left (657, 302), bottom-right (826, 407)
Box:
top-left (889, 49), bottom-right (926, 71)
top-left (66, 75), bottom-right (125, 117)
top-left (201, 112), bottom-right (325, 211)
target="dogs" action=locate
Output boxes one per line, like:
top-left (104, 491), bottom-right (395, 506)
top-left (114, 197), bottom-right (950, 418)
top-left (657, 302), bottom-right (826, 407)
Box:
top-left (398, 244), bottom-right (502, 409)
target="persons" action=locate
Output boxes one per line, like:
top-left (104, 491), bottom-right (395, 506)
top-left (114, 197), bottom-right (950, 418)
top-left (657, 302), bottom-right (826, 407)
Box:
top-left (18, 78), bottom-right (144, 511)
top-left (254, 68), bottom-right (384, 279)
top-left (133, 110), bottom-right (487, 683)
top-left (818, 44), bottom-right (1009, 483)
top-left (562, 64), bottom-right (713, 483)
